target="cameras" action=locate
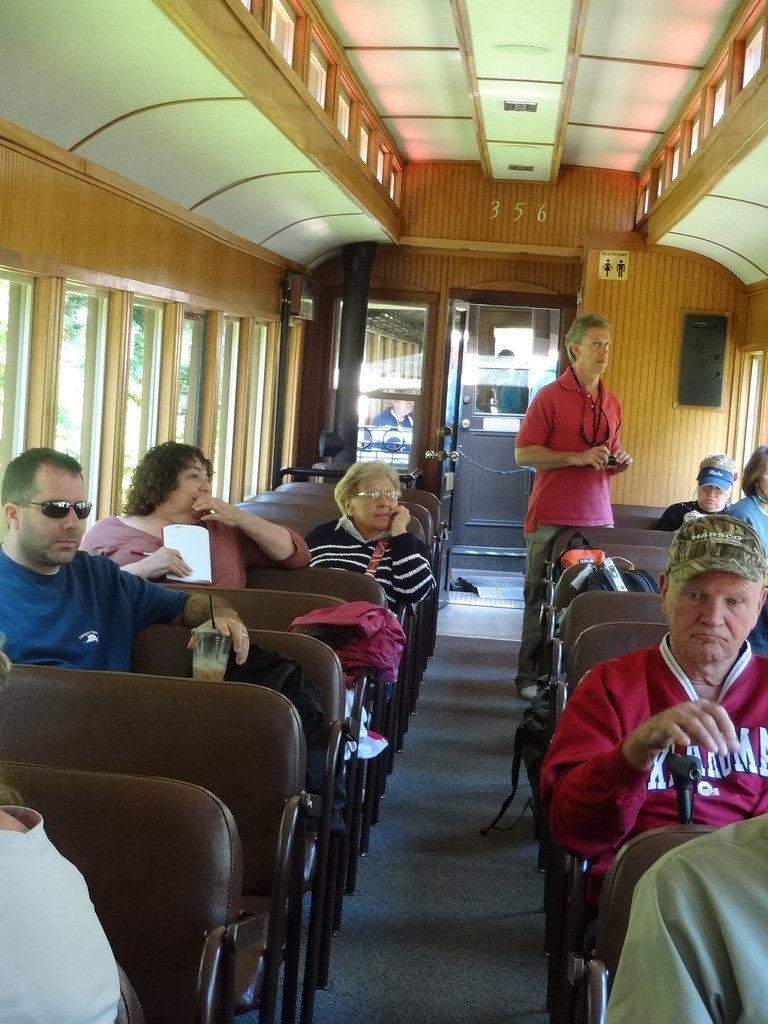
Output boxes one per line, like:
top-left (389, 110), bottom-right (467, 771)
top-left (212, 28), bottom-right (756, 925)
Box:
top-left (606, 456), bottom-right (620, 466)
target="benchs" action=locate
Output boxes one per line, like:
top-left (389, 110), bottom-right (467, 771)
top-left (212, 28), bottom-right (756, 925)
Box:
top-left (0, 483), bottom-right (766, 1021)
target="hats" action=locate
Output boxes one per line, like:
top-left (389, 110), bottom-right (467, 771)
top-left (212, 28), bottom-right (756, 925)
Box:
top-left (665, 515), bottom-right (766, 583)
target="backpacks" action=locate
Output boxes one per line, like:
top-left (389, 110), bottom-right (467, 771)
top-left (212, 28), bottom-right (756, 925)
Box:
top-left (579, 556), bottom-right (660, 592)
top-left (553, 532), bottom-right (606, 588)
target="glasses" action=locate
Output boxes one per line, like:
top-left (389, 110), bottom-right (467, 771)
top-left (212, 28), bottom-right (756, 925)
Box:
top-left (10, 499), bottom-right (92, 520)
top-left (580, 407), bottom-right (611, 448)
top-left (696, 467), bottom-right (736, 492)
top-left (349, 489), bottom-right (403, 503)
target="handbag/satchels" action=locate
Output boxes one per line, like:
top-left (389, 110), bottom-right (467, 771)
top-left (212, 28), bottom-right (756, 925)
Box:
top-left (480, 683), bottom-right (550, 847)
top-left (185, 643), bottom-right (324, 749)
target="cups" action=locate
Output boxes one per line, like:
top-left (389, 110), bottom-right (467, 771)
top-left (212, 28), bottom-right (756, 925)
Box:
top-left (190, 629), bottom-right (230, 682)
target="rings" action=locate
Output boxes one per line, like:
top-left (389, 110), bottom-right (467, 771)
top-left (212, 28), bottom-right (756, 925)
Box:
top-left (242, 634), bottom-right (247, 637)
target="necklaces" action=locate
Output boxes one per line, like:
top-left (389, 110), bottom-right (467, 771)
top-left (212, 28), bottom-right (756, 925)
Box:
top-left (756, 489), bottom-right (768, 504)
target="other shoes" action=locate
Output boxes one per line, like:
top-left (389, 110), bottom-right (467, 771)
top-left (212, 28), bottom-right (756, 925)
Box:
top-left (521, 685), bottom-right (537, 699)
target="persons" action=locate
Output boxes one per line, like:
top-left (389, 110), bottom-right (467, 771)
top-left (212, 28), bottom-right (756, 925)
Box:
top-left (540, 514), bottom-right (768, 1024)
top-left (78, 441), bottom-right (311, 590)
top-left (372, 389), bottom-right (414, 428)
top-left (486, 350), bottom-right (521, 414)
top-left (515, 314), bottom-right (633, 699)
top-left (0, 651), bottom-right (121, 1024)
top-left (722, 445), bottom-right (768, 575)
top-left (0, 447), bottom-right (250, 683)
top-left (656, 454), bottom-right (739, 531)
top-left (605, 813), bottom-right (768, 1024)
top-left (304, 460), bottom-right (437, 618)
